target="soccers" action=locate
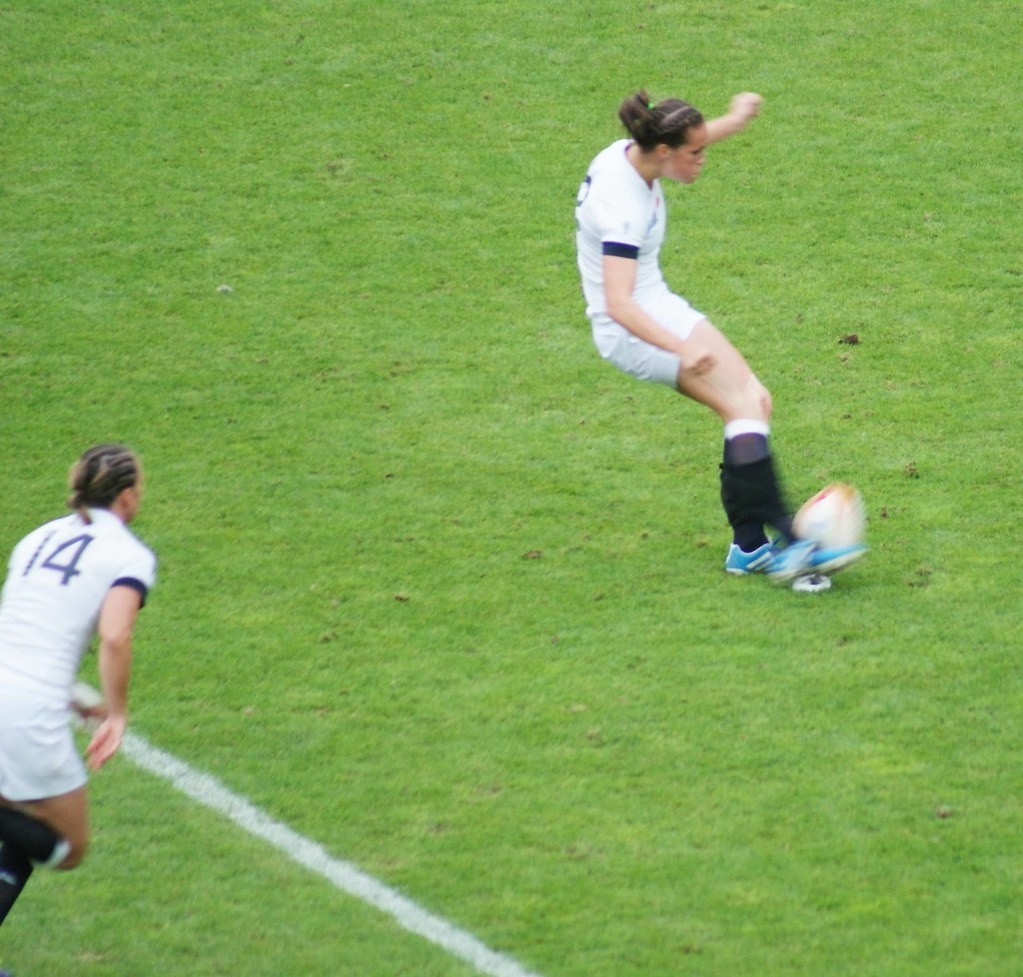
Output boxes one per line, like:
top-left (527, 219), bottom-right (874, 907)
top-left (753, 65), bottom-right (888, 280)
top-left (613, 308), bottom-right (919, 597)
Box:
top-left (792, 481), bottom-right (872, 546)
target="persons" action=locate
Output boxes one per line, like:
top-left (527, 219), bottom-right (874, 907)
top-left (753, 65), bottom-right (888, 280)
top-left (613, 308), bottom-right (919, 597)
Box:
top-left (0, 442), bottom-right (159, 977)
top-left (573, 90), bottom-right (872, 586)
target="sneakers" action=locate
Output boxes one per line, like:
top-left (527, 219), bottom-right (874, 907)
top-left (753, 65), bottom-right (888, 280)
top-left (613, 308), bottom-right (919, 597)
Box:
top-left (725, 540), bottom-right (830, 593)
top-left (769, 540), bottom-right (871, 584)
